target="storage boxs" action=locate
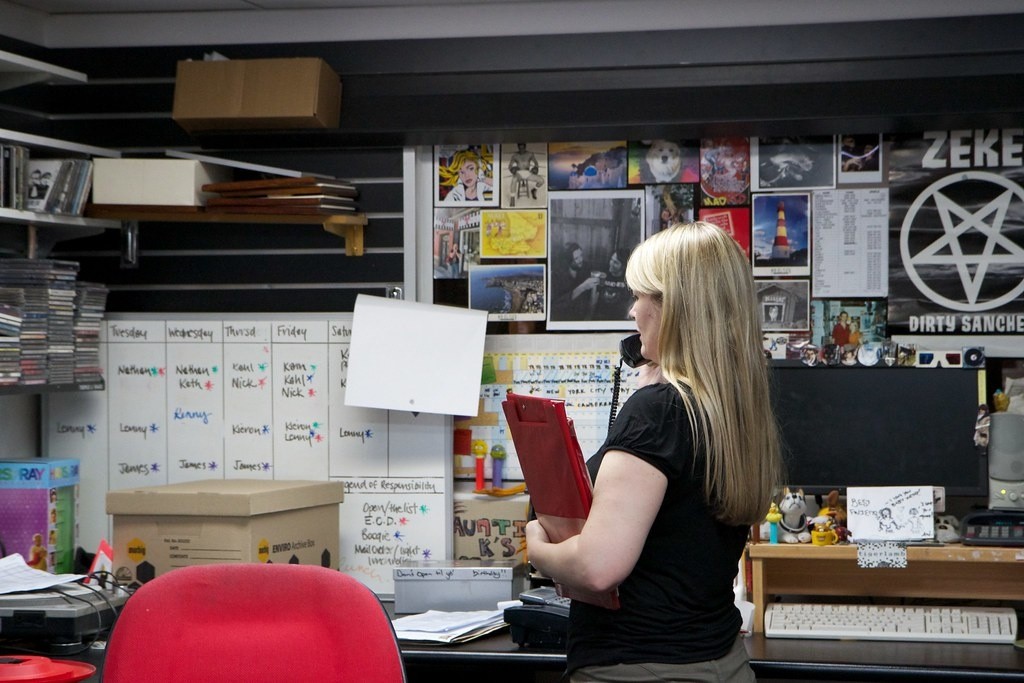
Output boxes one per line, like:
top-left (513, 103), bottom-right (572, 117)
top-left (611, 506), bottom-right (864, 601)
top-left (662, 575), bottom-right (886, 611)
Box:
top-left (0, 458), bottom-right (81, 576)
top-left (171, 58), bottom-right (342, 135)
top-left (105, 478), bottom-right (344, 590)
top-left (453, 490), bottom-right (532, 566)
top-left (392, 566), bottom-right (524, 615)
top-left (92, 158), bottom-right (228, 206)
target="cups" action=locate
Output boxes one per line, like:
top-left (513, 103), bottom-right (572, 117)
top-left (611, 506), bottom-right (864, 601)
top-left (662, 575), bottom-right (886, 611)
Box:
top-left (811, 530), bottom-right (838, 545)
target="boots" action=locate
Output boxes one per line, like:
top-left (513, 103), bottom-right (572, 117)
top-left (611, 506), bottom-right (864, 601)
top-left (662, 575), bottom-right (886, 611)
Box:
top-left (531, 188), bottom-right (538, 199)
top-left (510, 197), bottom-right (516, 207)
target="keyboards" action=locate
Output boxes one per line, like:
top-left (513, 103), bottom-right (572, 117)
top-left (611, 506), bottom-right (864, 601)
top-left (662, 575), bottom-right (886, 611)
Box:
top-left (764, 602), bottom-right (1018, 644)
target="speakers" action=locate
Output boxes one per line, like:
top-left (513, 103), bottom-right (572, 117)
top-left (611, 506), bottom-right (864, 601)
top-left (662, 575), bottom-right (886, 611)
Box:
top-left (988, 413), bottom-right (1024, 512)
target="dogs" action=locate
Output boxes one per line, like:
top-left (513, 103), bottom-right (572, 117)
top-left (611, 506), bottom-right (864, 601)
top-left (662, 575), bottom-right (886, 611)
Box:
top-left (768, 305), bottom-right (779, 322)
top-left (800, 344), bottom-right (819, 366)
top-left (644, 137), bottom-right (681, 183)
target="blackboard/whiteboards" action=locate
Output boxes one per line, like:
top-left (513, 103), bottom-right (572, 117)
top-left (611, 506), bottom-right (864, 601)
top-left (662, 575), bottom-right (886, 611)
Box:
top-left (38, 310), bottom-right (453, 603)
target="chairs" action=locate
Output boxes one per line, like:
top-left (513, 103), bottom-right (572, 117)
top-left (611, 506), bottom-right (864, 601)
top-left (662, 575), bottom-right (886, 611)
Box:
top-left (98, 562), bottom-right (407, 683)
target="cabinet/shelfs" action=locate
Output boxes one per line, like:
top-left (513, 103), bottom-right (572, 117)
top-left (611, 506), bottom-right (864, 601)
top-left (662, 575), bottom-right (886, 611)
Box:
top-left (0, 50), bottom-right (403, 394)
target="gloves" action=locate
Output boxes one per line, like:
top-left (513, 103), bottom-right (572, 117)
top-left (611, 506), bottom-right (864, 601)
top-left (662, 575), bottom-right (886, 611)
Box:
top-left (530, 166), bottom-right (538, 175)
top-left (510, 167), bottom-right (516, 175)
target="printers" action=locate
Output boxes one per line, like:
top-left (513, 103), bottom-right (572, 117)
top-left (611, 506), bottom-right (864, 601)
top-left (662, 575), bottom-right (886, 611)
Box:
top-left (0, 582), bottom-right (139, 683)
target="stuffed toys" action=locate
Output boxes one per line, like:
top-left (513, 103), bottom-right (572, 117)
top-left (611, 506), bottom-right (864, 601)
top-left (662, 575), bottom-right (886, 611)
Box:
top-left (760, 486), bottom-right (812, 544)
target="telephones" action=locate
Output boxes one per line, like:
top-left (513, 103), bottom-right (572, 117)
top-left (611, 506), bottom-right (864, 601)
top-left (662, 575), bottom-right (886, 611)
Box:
top-left (502, 587), bottom-right (570, 648)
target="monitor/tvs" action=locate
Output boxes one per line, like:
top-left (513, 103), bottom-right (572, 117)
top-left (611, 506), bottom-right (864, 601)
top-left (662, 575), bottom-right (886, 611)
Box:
top-left (768, 366), bottom-right (989, 498)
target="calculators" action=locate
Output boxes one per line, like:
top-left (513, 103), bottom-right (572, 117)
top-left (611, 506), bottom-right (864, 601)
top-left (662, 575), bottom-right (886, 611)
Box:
top-left (959, 510), bottom-right (1024, 547)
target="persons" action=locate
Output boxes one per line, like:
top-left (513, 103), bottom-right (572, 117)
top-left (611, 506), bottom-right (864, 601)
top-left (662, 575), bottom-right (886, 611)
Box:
top-left (848, 320), bottom-right (863, 346)
top-left (551, 241), bottom-right (599, 321)
top-left (832, 311), bottom-right (850, 347)
top-left (446, 243), bottom-right (461, 278)
top-left (524, 222), bottom-right (777, 683)
top-left (591, 248), bottom-right (635, 320)
